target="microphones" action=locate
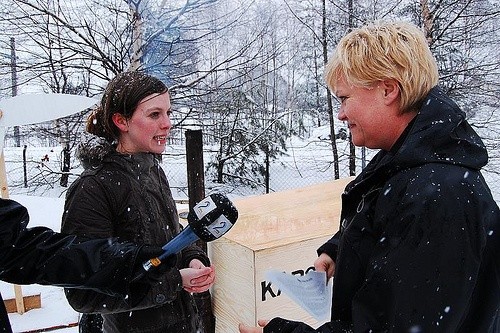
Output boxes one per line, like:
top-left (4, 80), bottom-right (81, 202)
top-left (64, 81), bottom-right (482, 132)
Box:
top-left (139, 194), bottom-right (238, 274)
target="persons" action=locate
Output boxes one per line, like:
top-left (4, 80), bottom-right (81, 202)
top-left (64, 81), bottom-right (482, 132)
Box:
top-left (0, 196), bottom-right (177, 333)
top-left (239, 21), bottom-right (500, 333)
top-left (61, 71), bottom-right (215, 333)
